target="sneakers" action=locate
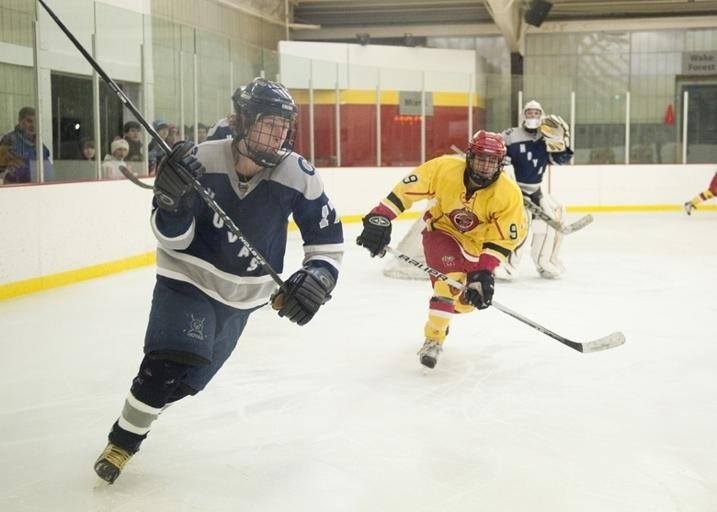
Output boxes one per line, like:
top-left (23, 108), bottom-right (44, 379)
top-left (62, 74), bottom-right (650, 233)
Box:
top-left (417, 337), bottom-right (445, 368)
top-left (686, 201), bottom-right (694, 215)
top-left (93, 441), bottom-right (134, 484)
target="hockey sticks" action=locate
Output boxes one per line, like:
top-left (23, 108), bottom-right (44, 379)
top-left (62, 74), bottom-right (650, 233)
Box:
top-left (119, 164), bottom-right (157, 190)
top-left (450, 145), bottom-right (593, 235)
top-left (386, 245), bottom-right (626, 355)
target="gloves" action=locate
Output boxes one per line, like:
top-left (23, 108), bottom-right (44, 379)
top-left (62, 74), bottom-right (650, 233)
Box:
top-left (357, 213), bottom-right (392, 259)
top-left (272, 259), bottom-right (339, 326)
top-left (153, 140), bottom-right (206, 216)
top-left (464, 269), bottom-right (494, 309)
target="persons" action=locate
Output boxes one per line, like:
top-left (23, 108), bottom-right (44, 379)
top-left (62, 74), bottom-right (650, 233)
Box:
top-left (93, 76), bottom-right (343, 485)
top-left (73, 118), bottom-right (207, 180)
top-left (0, 107), bottom-right (50, 184)
top-left (358, 129), bottom-right (529, 369)
top-left (494, 100), bottom-right (575, 280)
top-left (685, 171), bottom-right (716, 216)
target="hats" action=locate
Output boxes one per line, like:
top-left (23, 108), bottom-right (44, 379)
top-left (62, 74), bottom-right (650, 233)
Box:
top-left (153, 119), bottom-right (168, 131)
top-left (110, 135), bottom-right (130, 155)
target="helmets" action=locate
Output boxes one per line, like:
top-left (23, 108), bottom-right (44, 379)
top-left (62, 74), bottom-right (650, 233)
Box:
top-left (228, 76), bottom-right (298, 168)
top-left (520, 100), bottom-right (544, 131)
top-left (466, 130), bottom-right (507, 185)
